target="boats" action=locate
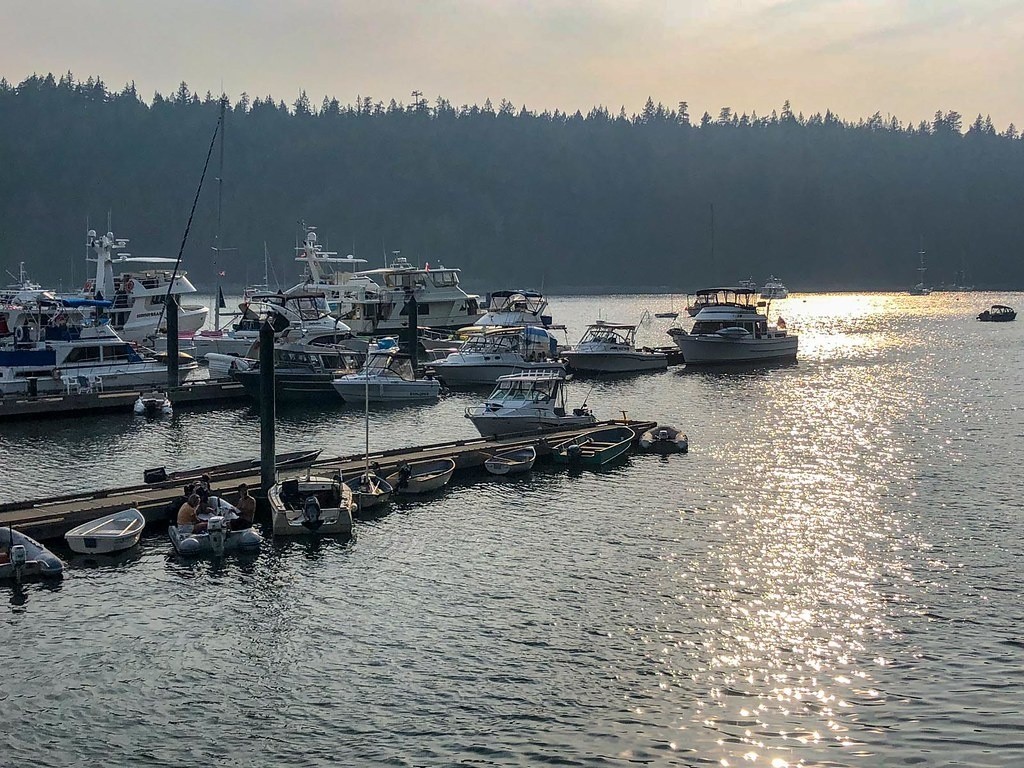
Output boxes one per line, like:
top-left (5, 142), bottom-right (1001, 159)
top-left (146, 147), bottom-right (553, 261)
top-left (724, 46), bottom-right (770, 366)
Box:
top-left (1, 96), bottom-right (571, 422)
top-left (0, 521), bottom-right (65, 583)
top-left (909, 282), bottom-right (934, 296)
top-left (637, 423), bottom-right (689, 457)
top-left (463, 349), bottom-right (601, 443)
top-left (267, 466), bottom-right (358, 537)
top-left (131, 390), bottom-right (174, 416)
top-left (384, 457), bottom-right (456, 495)
top-left (65, 508), bottom-right (145, 555)
top-left (167, 493), bottom-right (266, 557)
top-left (976, 305), bottom-right (1018, 322)
top-left (547, 424), bottom-right (636, 471)
top-left (560, 308), bottom-right (669, 375)
top-left (759, 274), bottom-right (789, 300)
top-left (665, 303), bottom-right (800, 367)
top-left (478, 443), bottom-right (537, 477)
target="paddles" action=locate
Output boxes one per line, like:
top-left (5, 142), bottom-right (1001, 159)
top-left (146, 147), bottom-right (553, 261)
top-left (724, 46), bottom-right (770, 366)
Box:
top-left (478, 451), bottom-right (514, 462)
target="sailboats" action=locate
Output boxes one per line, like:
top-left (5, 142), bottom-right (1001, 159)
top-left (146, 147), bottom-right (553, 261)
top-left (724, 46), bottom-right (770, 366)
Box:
top-left (338, 347), bottom-right (395, 512)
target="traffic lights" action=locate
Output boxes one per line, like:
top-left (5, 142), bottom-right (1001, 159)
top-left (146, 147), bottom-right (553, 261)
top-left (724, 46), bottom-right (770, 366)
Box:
top-left (685, 282), bottom-right (758, 316)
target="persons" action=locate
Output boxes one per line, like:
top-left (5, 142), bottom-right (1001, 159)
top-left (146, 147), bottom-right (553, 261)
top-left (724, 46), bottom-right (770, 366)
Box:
top-left (177, 494), bottom-right (207, 534)
top-left (230, 483), bottom-right (256, 531)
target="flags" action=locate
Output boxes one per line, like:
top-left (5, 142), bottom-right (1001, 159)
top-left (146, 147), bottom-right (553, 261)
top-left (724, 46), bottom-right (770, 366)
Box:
top-left (777, 317), bottom-right (785, 329)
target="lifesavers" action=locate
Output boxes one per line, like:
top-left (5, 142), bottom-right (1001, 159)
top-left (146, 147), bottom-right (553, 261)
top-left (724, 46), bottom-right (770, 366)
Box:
top-left (85, 282), bottom-right (91, 289)
top-left (15, 326), bottom-right (23, 341)
top-left (126, 281), bottom-right (134, 291)
